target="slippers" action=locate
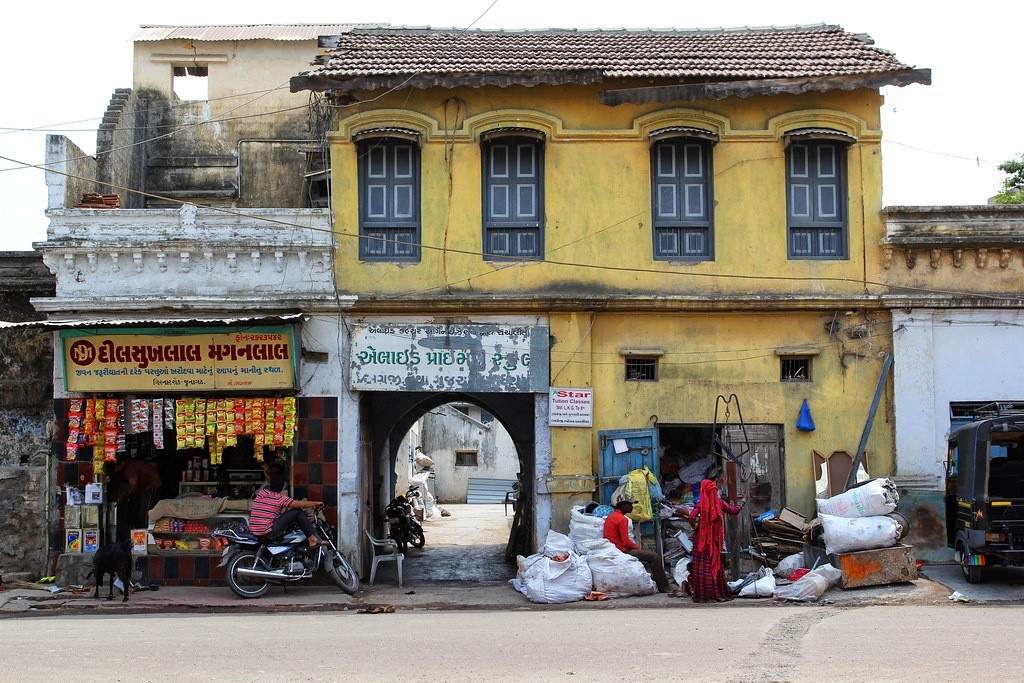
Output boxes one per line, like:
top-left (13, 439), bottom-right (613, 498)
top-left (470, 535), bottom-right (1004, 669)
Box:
top-left (310, 540), bottom-right (330, 550)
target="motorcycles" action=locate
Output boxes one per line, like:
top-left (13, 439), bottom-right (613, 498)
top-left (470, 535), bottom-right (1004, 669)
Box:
top-left (942, 400), bottom-right (1024, 583)
top-left (383, 484), bottom-right (425, 552)
top-left (211, 501), bottom-right (359, 599)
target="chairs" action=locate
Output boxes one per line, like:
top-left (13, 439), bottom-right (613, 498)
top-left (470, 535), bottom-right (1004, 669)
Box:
top-left (365, 530), bottom-right (405, 588)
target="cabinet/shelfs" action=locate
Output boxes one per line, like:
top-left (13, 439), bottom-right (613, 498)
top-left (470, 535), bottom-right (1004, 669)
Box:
top-left (148, 513), bottom-right (250, 554)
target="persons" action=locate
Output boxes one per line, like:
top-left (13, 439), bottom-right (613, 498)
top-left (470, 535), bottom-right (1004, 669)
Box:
top-left (603, 494), bottom-right (674, 593)
top-left (689, 480), bottom-right (748, 602)
top-left (249, 476), bottom-right (330, 548)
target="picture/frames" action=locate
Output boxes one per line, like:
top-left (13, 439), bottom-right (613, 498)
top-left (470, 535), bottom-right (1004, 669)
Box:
top-left (65, 529), bottom-right (82, 554)
top-left (83, 528), bottom-right (100, 552)
top-left (131, 530), bottom-right (147, 555)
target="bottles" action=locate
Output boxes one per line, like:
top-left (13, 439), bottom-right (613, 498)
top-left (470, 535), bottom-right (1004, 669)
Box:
top-left (70, 488), bottom-right (84, 504)
top-left (66, 482), bottom-right (74, 505)
top-left (170, 519), bottom-right (183, 532)
top-left (85, 482), bottom-right (102, 504)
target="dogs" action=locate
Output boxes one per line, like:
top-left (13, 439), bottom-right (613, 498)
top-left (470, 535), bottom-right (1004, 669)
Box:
top-left (92, 538), bottom-right (135, 603)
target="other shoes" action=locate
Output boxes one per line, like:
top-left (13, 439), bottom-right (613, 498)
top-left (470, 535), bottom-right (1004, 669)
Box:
top-left (661, 587), bottom-right (677, 593)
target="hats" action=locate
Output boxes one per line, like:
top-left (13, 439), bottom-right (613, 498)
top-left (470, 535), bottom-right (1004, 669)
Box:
top-left (617, 494), bottom-right (639, 504)
